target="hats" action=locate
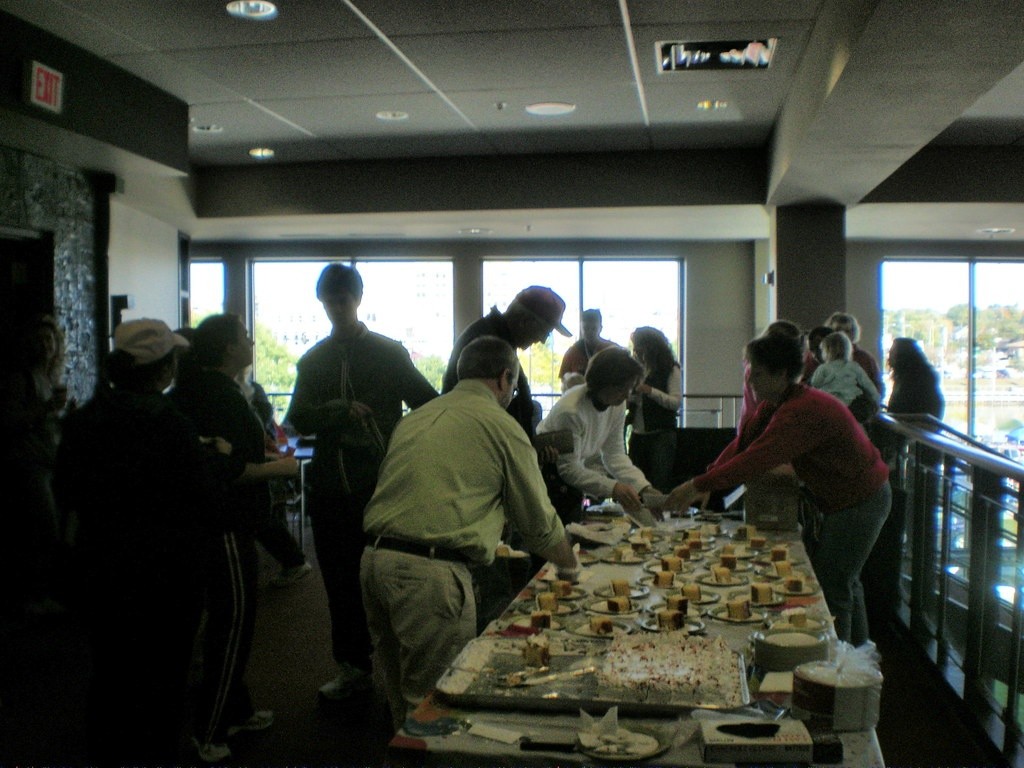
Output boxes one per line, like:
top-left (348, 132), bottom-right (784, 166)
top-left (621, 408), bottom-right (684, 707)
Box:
top-left (516, 286), bottom-right (572, 336)
top-left (114, 319), bottom-right (190, 364)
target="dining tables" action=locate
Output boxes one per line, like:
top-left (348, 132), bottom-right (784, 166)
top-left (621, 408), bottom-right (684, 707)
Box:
top-left (386, 503), bottom-right (889, 768)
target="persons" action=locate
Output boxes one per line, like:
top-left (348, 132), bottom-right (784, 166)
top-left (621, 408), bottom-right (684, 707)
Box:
top-left (663, 338), bottom-right (893, 662)
top-left (736, 312), bottom-right (942, 609)
top-left (360, 335), bottom-right (582, 734)
top-left (0, 280), bottom-right (313, 761)
top-left (288, 266), bottom-right (440, 711)
top-left (444, 290), bottom-right (682, 636)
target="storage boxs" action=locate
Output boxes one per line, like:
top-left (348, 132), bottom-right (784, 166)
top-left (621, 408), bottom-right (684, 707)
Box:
top-left (698, 718), bottom-right (815, 763)
top-left (742, 471), bottom-right (802, 533)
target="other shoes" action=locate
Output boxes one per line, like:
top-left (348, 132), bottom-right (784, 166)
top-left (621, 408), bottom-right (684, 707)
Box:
top-left (319, 663), bottom-right (371, 699)
top-left (271, 561), bottom-right (312, 587)
top-left (226, 710), bottom-right (274, 735)
top-left (191, 737), bottom-right (231, 762)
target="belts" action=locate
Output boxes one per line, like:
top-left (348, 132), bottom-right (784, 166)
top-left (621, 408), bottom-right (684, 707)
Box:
top-left (367, 535), bottom-right (468, 564)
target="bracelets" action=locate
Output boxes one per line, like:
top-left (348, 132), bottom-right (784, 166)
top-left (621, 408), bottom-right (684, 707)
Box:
top-left (551, 430), bottom-right (556, 445)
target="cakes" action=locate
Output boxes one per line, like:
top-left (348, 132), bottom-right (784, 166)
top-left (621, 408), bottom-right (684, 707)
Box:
top-left (598, 632), bottom-right (740, 707)
top-left (526, 530), bottom-right (808, 633)
top-left (523, 634), bottom-right (550, 666)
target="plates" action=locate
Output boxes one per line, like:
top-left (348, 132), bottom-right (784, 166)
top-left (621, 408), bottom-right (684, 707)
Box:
top-left (583, 598), bottom-right (644, 614)
top-left (663, 588), bottom-right (721, 604)
top-left (643, 562), bottom-right (694, 575)
top-left (758, 567), bottom-right (811, 579)
top-left (640, 617), bottom-right (706, 635)
top-left (646, 604), bottom-right (707, 617)
top-left (511, 617), bottom-right (566, 630)
top-left (734, 591), bottom-right (785, 606)
top-left (532, 586), bottom-right (588, 600)
top-left (708, 605), bottom-right (764, 623)
top-left (518, 598), bottom-right (579, 616)
top-left (751, 554), bottom-right (806, 566)
top-left (694, 571), bottom-right (750, 587)
top-left (576, 550), bottom-right (599, 564)
top-left (635, 575), bottom-right (689, 591)
top-left (594, 584), bottom-right (650, 598)
top-left (792, 662), bottom-right (883, 732)
top-left (568, 621), bottom-right (634, 640)
top-left (613, 525), bottom-right (789, 559)
top-left (601, 553), bottom-right (647, 563)
top-left (772, 582), bottom-right (821, 596)
top-left (703, 559), bottom-right (753, 572)
top-left (577, 722), bottom-right (672, 760)
top-left (767, 617), bottom-right (830, 634)
top-left (752, 629), bottom-right (830, 681)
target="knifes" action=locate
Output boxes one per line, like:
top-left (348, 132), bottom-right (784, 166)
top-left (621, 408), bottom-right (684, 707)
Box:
top-left (520, 741), bottom-right (595, 752)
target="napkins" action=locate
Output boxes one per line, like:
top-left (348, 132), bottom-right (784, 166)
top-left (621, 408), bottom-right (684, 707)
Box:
top-left (579, 704), bottom-right (632, 753)
top-left (467, 723), bottom-right (523, 746)
top-left (759, 671), bottom-right (795, 694)
